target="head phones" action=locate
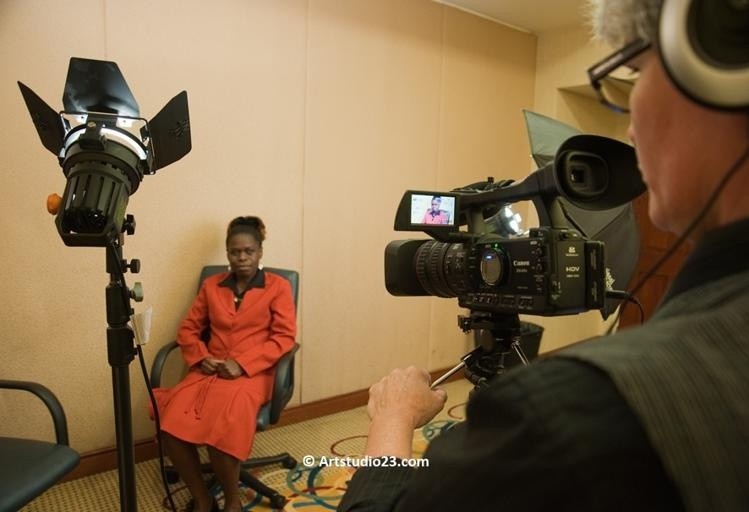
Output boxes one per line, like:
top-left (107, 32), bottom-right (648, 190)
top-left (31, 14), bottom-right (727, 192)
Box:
top-left (658, 0), bottom-right (747, 111)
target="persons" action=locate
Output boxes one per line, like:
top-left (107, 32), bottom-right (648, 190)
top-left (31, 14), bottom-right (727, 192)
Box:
top-left (334, 0), bottom-right (748, 510)
top-left (148, 215), bottom-right (298, 511)
top-left (421, 195), bottom-right (449, 224)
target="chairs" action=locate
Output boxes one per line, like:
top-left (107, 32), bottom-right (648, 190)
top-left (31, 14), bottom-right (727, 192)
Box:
top-left (1, 381), bottom-right (81, 512)
top-left (150, 265), bottom-right (302, 512)
top-left (426, 208), bottom-right (451, 225)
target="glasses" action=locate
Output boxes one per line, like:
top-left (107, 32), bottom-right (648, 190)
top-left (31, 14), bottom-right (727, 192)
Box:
top-left (587, 39), bottom-right (652, 113)
